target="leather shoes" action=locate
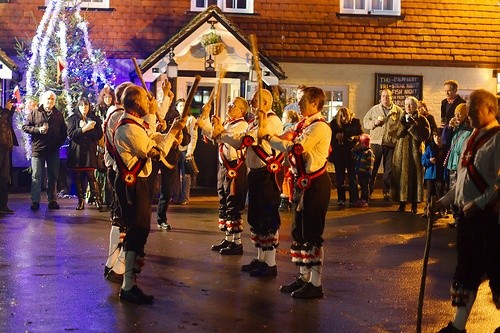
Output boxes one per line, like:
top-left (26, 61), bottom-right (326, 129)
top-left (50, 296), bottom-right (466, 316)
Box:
top-left (104, 266), bottom-right (123, 284)
top-left (250, 263), bottom-right (277, 277)
top-left (211, 239), bottom-right (231, 250)
top-left (220, 242), bottom-right (243, 255)
top-left (278, 277), bottom-right (307, 292)
top-left (241, 259), bottom-right (263, 270)
top-left (119, 285), bottom-right (154, 302)
top-left (291, 282), bottom-right (323, 298)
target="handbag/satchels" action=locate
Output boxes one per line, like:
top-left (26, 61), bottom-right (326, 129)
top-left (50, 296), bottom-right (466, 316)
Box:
top-left (327, 150), bottom-right (337, 163)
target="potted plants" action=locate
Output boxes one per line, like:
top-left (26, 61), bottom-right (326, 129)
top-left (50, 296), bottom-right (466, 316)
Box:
top-left (201, 33), bottom-right (225, 55)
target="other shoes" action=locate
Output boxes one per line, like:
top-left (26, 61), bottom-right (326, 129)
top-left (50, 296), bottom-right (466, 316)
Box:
top-left (180, 201), bottom-right (189, 205)
top-left (384, 192), bottom-right (390, 200)
top-left (338, 200), bottom-right (344, 205)
top-left (157, 223), bottom-right (170, 230)
top-left (48, 201), bottom-right (60, 209)
top-left (30, 202), bottom-right (39, 210)
top-left (436, 321), bottom-right (466, 333)
top-left (0, 208), bottom-right (14, 215)
top-left (411, 205), bottom-right (418, 213)
top-left (422, 209), bottom-right (426, 217)
top-left (438, 211), bottom-right (449, 218)
top-left (279, 198), bottom-right (289, 210)
top-left (398, 205), bottom-right (405, 211)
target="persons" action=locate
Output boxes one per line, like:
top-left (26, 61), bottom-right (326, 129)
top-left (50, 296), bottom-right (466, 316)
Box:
top-left (0, 80), bottom-right (500, 333)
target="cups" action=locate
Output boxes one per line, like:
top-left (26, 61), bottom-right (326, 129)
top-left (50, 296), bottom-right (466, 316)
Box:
top-left (88, 121), bottom-right (95, 128)
top-left (405, 113), bottom-right (412, 122)
top-left (43, 122), bottom-right (48, 132)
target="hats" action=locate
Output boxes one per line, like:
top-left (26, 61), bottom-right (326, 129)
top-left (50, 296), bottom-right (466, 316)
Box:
top-left (360, 133), bottom-right (370, 148)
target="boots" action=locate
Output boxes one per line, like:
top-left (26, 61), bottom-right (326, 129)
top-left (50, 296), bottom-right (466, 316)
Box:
top-left (76, 184), bottom-right (84, 209)
top-left (92, 182), bottom-right (103, 211)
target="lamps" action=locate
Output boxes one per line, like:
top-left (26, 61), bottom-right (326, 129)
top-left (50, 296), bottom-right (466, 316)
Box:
top-left (167, 47), bottom-right (178, 78)
top-left (249, 56), bottom-right (262, 82)
